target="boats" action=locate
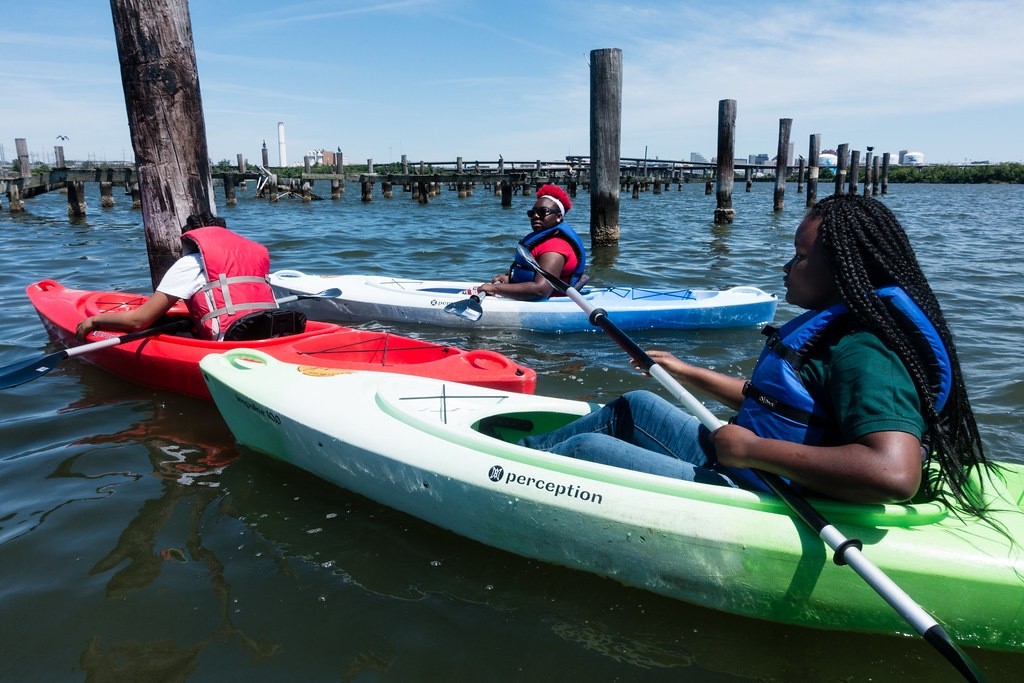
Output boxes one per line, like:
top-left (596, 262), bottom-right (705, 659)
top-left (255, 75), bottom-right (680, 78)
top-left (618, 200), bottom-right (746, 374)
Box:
top-left (198, 346), bottom-right (1024, 656)
top-left (26, 281), bottom-right (537, 406)
top-left (266, 267), bottom-right (778, 334)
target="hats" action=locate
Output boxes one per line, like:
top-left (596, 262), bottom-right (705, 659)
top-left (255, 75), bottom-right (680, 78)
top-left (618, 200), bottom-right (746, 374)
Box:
top-left (537, 184), bottom-right (571, 217)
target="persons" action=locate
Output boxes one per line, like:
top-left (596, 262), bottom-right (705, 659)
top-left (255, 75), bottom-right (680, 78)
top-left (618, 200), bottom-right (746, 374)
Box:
top-left (75, 213), bottom-right (279, 342)
top-left (517, 194), bottom-right (993, 505)
top-left (458, 185), bottom-right (585, 299)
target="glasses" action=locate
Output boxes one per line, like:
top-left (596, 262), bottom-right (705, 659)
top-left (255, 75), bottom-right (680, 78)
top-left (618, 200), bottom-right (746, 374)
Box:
top-left (527, 208), bottom-right (560, 218)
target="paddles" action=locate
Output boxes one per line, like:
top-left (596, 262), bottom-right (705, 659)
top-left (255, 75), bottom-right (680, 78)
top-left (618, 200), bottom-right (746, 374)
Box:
top-left (516, 242), bottom-right (989, 683)
top-left (443, 280), bottom-right (502, 323)
top-left (0, 288), bottom-right (342, 389)
